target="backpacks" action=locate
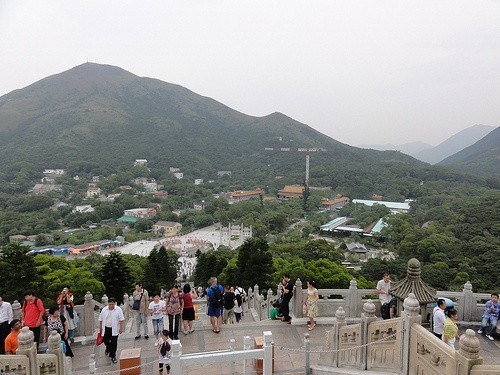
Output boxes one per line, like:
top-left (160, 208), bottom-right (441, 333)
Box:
top-left (237, 288), bottom-right (245, 303)
top-left (210, 287), bottom-right (223, 307)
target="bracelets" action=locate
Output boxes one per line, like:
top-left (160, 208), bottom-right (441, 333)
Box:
top-left (385, 292), bottom-right (386, 294)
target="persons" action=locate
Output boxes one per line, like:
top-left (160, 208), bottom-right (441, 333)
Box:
top-left (154, 329), bottom-right (172, 375)
top-left (376, 271), bottom-right (396, 333)
top-left (5, 319), bottom-right (22, 355)
top-left (304, 279), bottom-right (319, 331)
top-left (56, 285), bottom-right (74, 313)
top-left (269, 303), bottom-right (284, 320)
top-left (196, 287), bottom-right (205, 298)
top-left (63, 303), bottom-right (80, 346)
top-left (0, 295), bottom-right (13, 355)
top-left (166, 284), bottom-right (197, 340)
top-left (45, 327), bottom-right (66, 353)
top-left (98, 297), bottom-right (125, 364)
top-left (22, 291), bottom-right (44, 354)
top-left (433, 297), bottom-right (460, 349)
top-left (477, 293), bottom-right (500, 341)
top-left (205, 277), bottom-right (247, 334)
top-left (148, 294), bottom-right (166, 340)
top-left (43, 306), bottom-right (74, 358)
top-left (132, 282), bottom-right (149, 340)
top-left (280, 273), bottom-right (293, 324)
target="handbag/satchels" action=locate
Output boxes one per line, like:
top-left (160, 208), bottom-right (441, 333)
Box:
top-left (96, 333), bottom-right (102, 345)
top-left (302, 304), bottom-right (307, 315)
top-left (132, 300), bottom-right (139, 310)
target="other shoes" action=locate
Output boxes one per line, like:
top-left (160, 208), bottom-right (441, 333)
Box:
top-left (145, 335), bottom-right (149, 339)
top-left (112, 357), bottom-right (117, 363)
top-left (183, 331), bottom-right (188, 335)
top-left (478, 330), bottom-right (483, 334)
top-left (486, 334), bottom-right (494, 341)
top-left (308, 323), bottom-right (315, 330)
top-left (135, 335), bottom-right (141, 339)
top-left (213, 328), bottom-right (219, 334)
top-left (288, 316), bottom-right (292, 324)
top-left (189, 330), bottom-right (194, 334)
top-left (105, 349), bottom-right (109, 355)
